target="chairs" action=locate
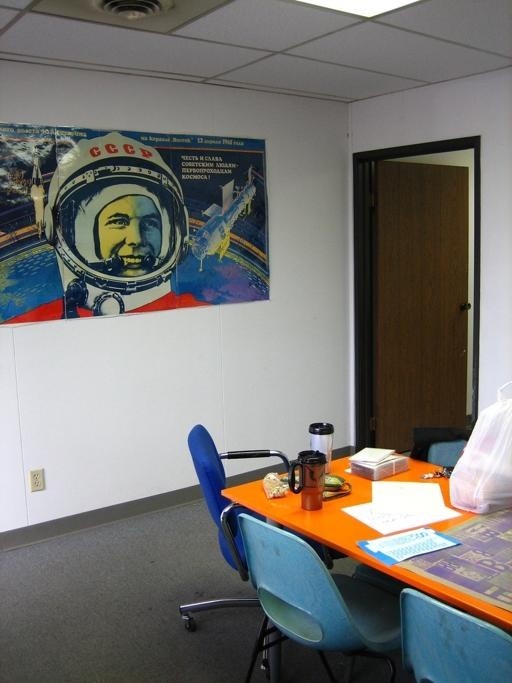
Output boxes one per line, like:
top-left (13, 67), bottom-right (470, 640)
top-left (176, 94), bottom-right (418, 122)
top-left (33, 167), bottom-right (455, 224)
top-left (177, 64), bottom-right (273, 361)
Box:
top-left (178, 424), bottom-right (511, 682)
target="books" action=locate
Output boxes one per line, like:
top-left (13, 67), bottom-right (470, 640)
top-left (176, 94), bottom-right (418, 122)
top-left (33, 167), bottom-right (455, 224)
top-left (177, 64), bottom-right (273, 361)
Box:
top-left (350, 443), bottom-right (414, 478)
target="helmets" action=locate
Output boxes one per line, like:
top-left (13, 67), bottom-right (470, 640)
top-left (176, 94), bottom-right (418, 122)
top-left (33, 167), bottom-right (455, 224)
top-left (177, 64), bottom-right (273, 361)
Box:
top-left (40, 131), bottom-right (190, 315)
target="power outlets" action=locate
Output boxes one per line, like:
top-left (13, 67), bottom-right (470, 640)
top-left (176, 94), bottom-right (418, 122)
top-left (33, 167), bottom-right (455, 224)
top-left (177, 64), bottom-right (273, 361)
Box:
top-left (28, 467), bottom-right (46, 493)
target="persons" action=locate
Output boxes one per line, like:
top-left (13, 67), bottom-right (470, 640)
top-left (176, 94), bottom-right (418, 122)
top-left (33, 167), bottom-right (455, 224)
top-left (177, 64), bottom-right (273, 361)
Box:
top-left (0, 130), bottom-right (229, 325)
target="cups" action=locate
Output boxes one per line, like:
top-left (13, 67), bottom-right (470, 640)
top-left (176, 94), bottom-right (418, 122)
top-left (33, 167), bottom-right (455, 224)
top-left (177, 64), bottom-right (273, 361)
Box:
top-left (308, 421), bottom-right (335, 474)
top-left (287, 449), bottom-right (327, 511)
